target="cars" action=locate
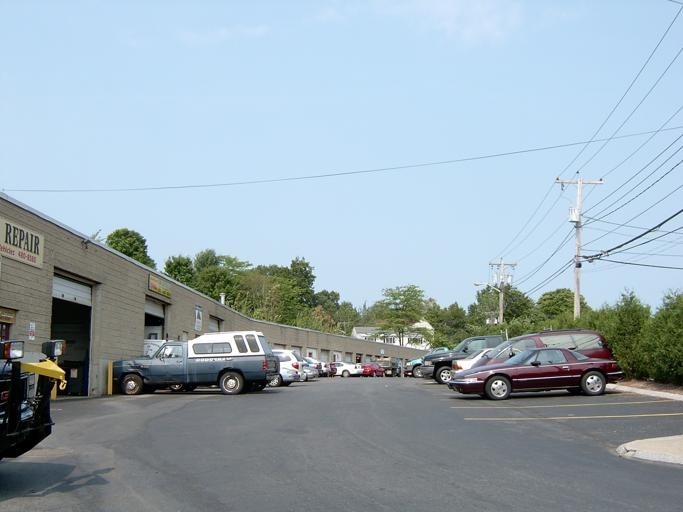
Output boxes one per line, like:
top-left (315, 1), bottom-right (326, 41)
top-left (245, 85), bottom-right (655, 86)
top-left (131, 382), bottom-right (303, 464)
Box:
top-left (445, 345), bottom-right (626, 401)
top-left (356, 360), bottom-right (385, 377)
top-left (448, 348), bottom-right (497, 381)
top-left (301, 357), bottom-right (323, 379)
top-left (322, 360), bottom-right (336, 375)
top-left (311, 358), bottom-right (328, 375)
top-left (330, 361), bottom-right (364, 377)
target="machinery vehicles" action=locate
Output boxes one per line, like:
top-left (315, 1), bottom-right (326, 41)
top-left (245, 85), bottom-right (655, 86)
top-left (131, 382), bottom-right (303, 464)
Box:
top-left (373, 356), bottom-right (401, 377)
top-left (0, 333), bottom-right (68, 460)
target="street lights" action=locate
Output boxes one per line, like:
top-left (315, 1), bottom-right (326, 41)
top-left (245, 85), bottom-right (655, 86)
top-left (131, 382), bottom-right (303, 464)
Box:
top-left (471, 280), bottom-right (504, 324)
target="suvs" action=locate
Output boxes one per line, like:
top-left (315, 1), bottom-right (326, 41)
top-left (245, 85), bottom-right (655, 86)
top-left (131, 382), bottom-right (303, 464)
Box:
top-left (264, 347), bottom-right (309, 388)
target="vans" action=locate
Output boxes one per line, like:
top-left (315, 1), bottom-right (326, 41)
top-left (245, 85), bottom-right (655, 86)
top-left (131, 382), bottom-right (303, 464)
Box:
top-left (467, 325), bottom-right (614, 371)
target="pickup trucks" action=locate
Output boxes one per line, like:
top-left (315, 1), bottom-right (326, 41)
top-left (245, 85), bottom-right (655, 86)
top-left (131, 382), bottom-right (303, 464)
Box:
top-left (110, 328), bottom-right (282, 396)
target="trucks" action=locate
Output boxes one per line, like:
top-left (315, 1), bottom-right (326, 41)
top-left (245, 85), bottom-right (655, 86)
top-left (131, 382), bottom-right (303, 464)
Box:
top-left (403, 347), bottom-right (454, 378)
top-left (418, 333), bottom-right (504, 385)
